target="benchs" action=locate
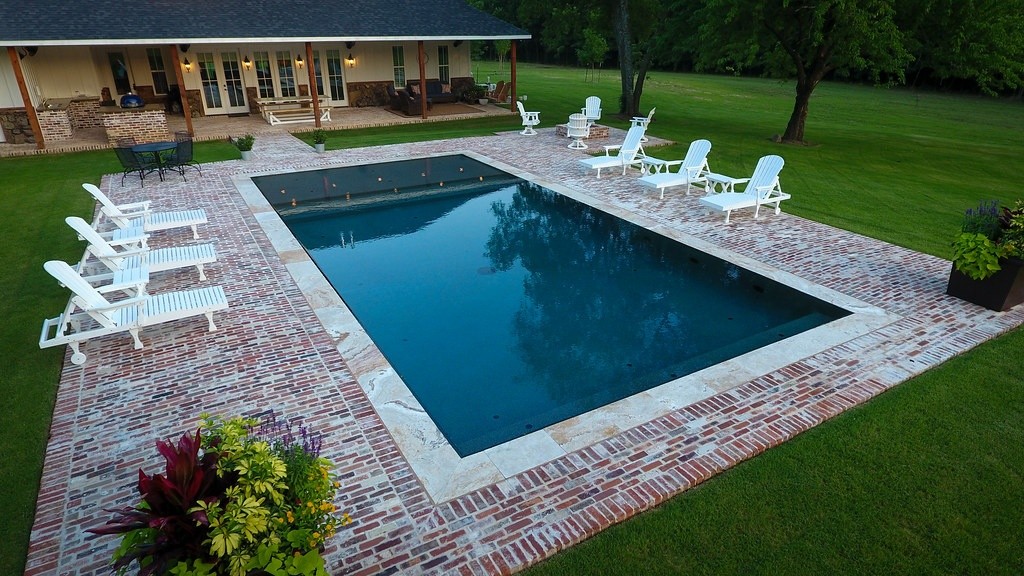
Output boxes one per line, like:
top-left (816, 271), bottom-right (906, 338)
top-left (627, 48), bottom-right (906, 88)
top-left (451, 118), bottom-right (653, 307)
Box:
top-left (256, 102), bottom-right (332, 127)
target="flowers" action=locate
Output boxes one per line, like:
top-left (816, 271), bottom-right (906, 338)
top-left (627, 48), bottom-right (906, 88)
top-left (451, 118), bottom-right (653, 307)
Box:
top-left (82, 411), bottom-right (352, 576)
top-left (948, 194), bottom-right (1024, 280)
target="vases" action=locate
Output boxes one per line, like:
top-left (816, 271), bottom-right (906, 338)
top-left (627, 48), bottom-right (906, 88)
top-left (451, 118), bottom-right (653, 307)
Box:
top-left (947, 252), bottom-right (1024, 311)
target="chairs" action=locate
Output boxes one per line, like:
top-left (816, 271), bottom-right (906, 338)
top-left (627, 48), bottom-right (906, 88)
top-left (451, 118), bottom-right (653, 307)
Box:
top-left (565, 96), bottom-right (791, 223)
top-left (485, 81), bottom-right (540, 135)
top-left (39, 137), bottom-right (230, 365)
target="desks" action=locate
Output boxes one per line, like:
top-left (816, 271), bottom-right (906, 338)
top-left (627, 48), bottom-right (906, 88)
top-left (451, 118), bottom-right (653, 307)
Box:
top-left (253, 95), bottom-right (331, 124)
top-left (113, 227), bottom-right (144, 250)
top-left (133, 143), bottom-right (177, 181)
top-left (114, 267), bottom-right (149, 297)
top-left (705, 174), bottom-right (735, 193)
top-left (642, 158), bottom-right (665, 175)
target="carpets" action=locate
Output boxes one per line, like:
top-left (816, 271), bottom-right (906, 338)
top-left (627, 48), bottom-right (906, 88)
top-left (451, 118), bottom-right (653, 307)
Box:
top-left (384, 103), bottom-right (486, 118)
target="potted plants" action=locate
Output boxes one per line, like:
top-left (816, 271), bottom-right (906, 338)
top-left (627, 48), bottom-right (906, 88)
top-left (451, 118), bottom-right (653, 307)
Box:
top-left (464, 85), bottom-right (488, 105)
top-left (312, 129), bottom-right (328, 153)
top-left (235, 132), bottom-right (255, 161)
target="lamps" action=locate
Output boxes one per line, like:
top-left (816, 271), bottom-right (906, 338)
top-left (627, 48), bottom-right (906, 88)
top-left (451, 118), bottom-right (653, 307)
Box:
top-left (184, 57), bottom-right (191, 73)
top-left (244, 56), bottom-right (250, 70)
top-left (348, 53), bottom-right (355, 67)
top-left (296, 54), bottom-right (303, 68)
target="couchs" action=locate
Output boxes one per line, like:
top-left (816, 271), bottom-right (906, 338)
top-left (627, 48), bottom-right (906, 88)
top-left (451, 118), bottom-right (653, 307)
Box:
top-left (387, 80), bottom-right (458, 116)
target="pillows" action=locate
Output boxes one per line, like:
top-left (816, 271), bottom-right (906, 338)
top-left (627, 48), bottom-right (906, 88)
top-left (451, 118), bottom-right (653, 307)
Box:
top-left (441, 83), bottom-right (451, 93)
top-left (410, 85), bottom-right (420, 94)
